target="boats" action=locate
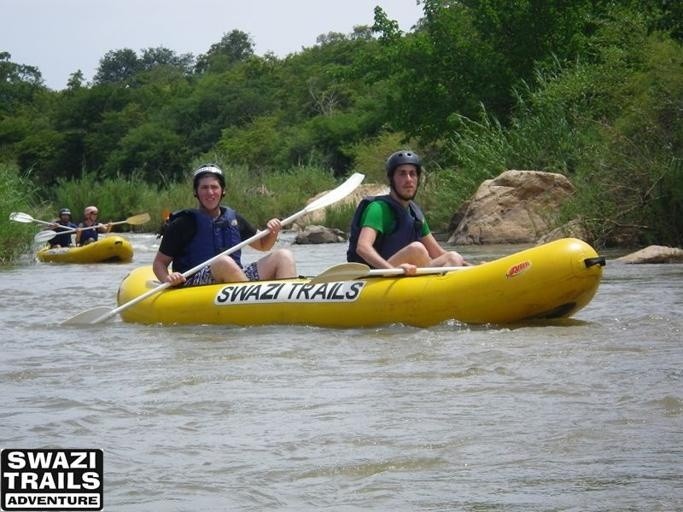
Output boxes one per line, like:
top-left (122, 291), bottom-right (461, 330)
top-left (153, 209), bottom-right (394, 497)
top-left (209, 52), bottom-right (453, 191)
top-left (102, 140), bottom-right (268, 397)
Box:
top-left (112, 235), bottom-right (607, 334)
top-left (35, 235), bottom-right (136, 264)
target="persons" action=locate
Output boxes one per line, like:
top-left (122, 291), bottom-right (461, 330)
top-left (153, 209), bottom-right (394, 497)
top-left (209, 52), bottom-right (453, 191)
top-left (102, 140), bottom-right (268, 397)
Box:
top-left (43, 207), bottom-right (79, 249)
top-left (75, 205), bottom-right (112, 247)
top-left (152, 163), bottom-right (296, 289)
top-left (346, 149), bottom-right (464, 276)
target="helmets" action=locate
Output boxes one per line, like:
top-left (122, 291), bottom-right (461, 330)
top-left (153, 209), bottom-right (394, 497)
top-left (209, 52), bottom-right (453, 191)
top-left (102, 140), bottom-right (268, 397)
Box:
top-left (84, 206), bottom-right (98, 217)
top-left (387, 150), bottom-right (422, 175)
top-left (193, 164), bottom-right (225, 187)
top-left (59, 208), bottom-right (71, 218)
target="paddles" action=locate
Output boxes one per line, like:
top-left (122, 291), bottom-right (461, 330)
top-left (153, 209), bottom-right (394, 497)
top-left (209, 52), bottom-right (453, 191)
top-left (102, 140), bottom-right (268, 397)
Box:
top-left (35, 212), bottom-right (151, 242)
top-left (9, 212), bottom-right (75, 230)
top-left (306, 262), bottom-right (470, 286)
top-left (59, 173), bottom-right (365, 325)
